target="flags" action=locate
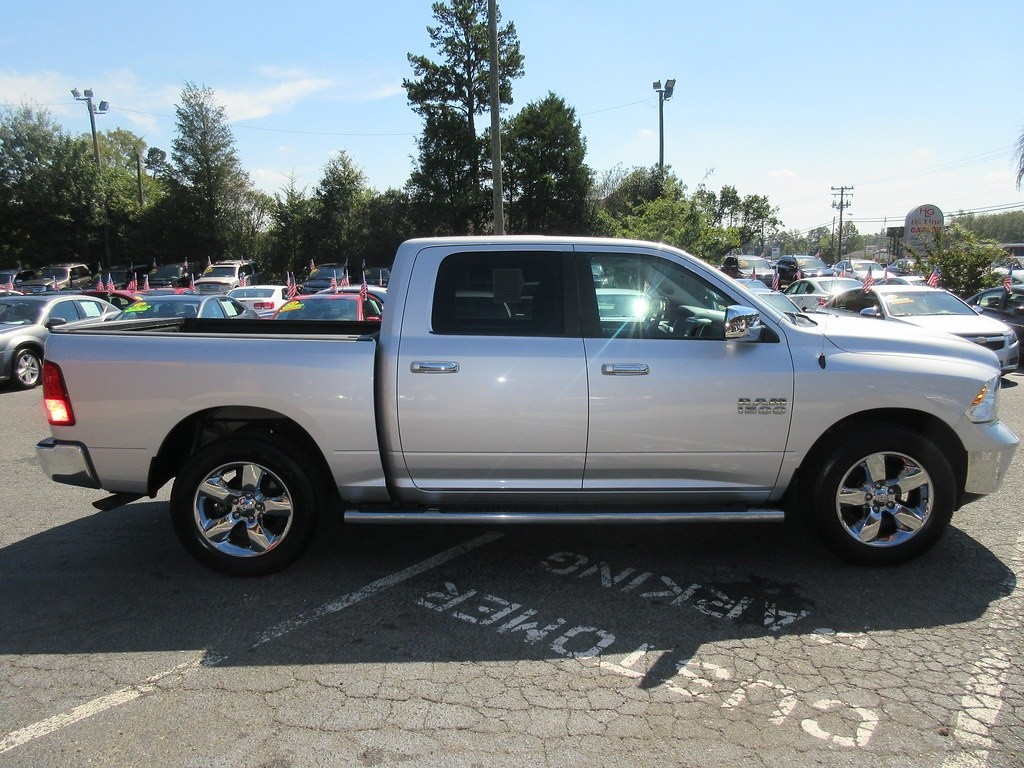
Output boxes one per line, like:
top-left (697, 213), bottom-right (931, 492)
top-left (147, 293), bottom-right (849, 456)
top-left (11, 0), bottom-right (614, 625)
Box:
top-left (54, 276), bottom-right (59, 292)
top-left (750, 251), bottom-right (889, 294)
top-left (285, 258), bottom-right (382, 301)
top-left (1011, 249), bottom-right (1014, 261)
top-left (98, 255), bottom-right (243, 271)
top-left (239, 272), bottom-right (246, 287)
top-left (190, 274), bottom-right (196, 292)
top-left (926, 266), bottom-right (941, 288)
top-left (0, 276), bottom-right (14, 290)
top-left (1003, 265), bottom-right (1013, 295)
top-left (97, 273), bottom-right (150, 296)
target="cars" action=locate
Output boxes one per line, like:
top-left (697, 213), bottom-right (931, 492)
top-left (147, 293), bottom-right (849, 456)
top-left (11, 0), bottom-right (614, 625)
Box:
top-left (0, 254), bottom-right (1023, 391)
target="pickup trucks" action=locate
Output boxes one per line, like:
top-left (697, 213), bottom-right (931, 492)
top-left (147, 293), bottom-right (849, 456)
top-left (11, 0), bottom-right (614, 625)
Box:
top-left (35, 234), bottom-right (1019, 579)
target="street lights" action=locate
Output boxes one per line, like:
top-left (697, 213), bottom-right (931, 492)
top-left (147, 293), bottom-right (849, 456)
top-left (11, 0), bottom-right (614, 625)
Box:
top-left (653, 80), bottom-right (676, 175)
top-left (71, 86), bottom-right (110, 272)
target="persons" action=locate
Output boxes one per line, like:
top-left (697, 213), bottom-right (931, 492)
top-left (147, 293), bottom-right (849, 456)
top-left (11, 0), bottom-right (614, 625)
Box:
top-left (717, 257), bottom-right (743, 279)
top-left (730, 318), bottom-right (746, 331)
